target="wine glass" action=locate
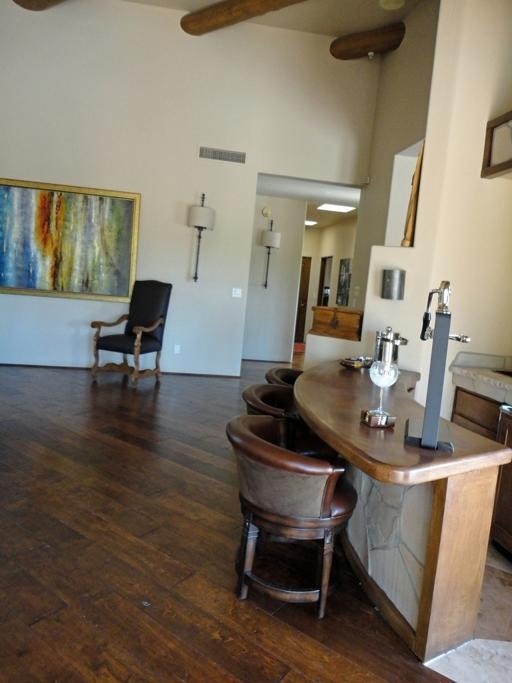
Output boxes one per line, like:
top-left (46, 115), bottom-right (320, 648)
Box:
top-left (367, 360), bottom-right (399, 417)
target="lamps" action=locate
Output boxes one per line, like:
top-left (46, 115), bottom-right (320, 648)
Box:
top-left (185, 190), bottom-right (215, 280)
top-left (258, 218), bottom-right (281, 290)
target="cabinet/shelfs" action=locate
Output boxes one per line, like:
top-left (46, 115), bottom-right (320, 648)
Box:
top-left (488, 410), bottom-right (511, 552)
top-left (451, 386), bottom-right (499, 441)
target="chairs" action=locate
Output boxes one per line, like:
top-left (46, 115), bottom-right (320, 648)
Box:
top-left (224, 365), bottom-right (355, 621)
top-left (90, 278), bottom-right (172, 388)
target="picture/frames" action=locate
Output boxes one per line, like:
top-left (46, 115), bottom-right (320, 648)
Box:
top-left (0, 177), bottom-right (139, 303)
